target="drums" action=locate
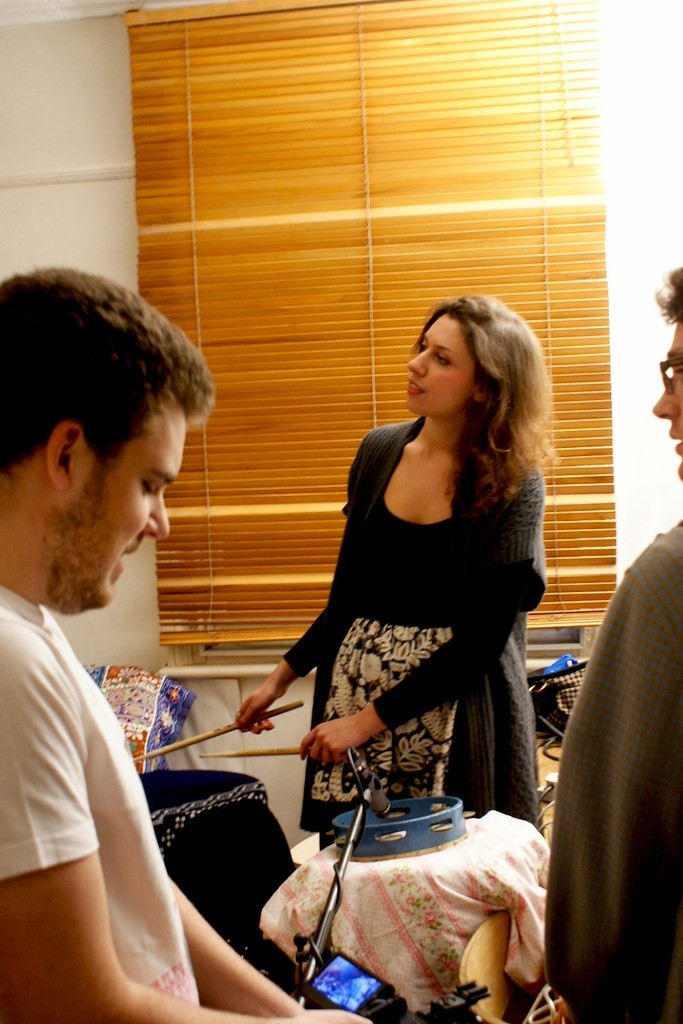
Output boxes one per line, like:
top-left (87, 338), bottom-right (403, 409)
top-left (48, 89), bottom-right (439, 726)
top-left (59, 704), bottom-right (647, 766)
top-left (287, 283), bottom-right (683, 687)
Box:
top-left (139, 769), bottom-right (269, 860)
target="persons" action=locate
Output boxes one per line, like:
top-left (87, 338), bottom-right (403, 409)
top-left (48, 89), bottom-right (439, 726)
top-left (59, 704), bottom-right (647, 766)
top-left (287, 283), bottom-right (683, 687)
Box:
top-left (235, 294), bottom-right (553, 852)
top-left (0, 266), bottom-right (375, 1024)
top-left (544, 266), bottom-right (683, 1024)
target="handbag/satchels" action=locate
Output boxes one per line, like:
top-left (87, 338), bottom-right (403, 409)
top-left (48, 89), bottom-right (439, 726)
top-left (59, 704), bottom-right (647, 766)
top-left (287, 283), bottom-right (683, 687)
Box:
top-left (529, 656), bottom-right (587, 735)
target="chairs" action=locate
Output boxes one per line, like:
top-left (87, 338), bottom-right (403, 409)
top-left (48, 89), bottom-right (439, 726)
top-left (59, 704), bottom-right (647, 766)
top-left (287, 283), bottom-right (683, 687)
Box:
top-left (459, 913), bottom-right (514, 1024)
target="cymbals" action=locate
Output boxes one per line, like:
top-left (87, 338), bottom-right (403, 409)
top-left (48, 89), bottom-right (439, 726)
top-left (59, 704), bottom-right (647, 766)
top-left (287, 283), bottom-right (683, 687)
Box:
top-left (332, 794), bottom-right (477, 863)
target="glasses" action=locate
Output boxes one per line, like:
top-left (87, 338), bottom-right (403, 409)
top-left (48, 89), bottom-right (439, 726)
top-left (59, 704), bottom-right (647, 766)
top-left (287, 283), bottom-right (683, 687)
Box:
top-left (660, 357), bottom-right (683, 394)
